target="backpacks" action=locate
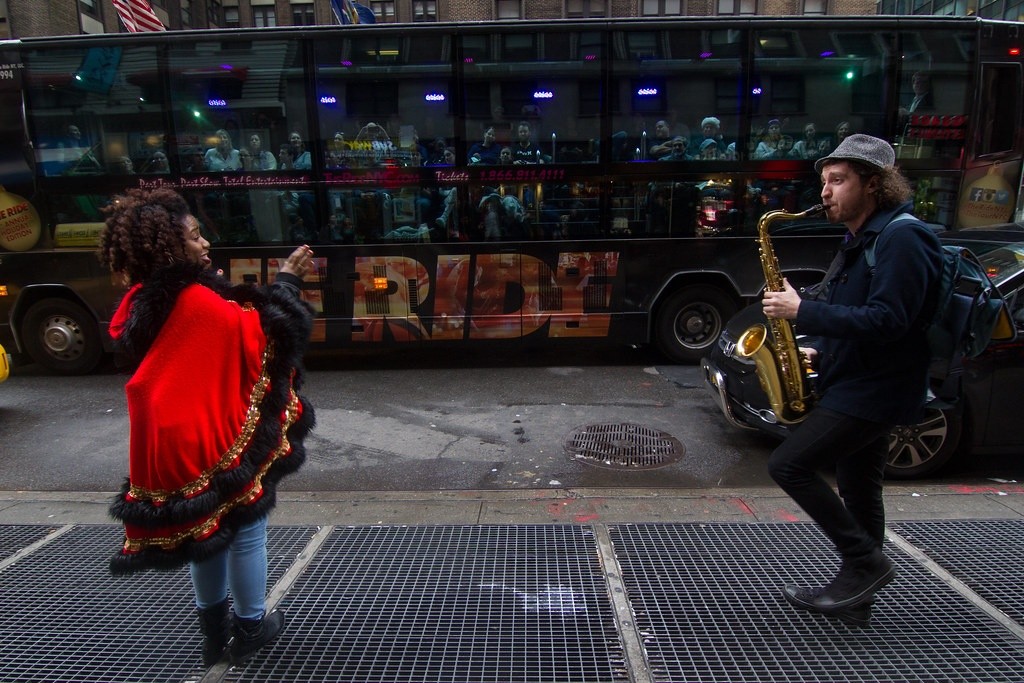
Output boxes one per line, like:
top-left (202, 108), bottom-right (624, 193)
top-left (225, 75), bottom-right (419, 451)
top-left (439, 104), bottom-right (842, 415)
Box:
top-left (866, 213), bottom-right (1017, 380)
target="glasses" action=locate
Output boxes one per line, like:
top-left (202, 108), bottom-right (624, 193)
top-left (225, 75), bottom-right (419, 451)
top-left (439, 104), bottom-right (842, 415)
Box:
top-left (279, 150), bottom-right (288, 156)
top-left (672, 143), bottom-right (683, 148)
top-left (839, 127), bottom-right (849, 131)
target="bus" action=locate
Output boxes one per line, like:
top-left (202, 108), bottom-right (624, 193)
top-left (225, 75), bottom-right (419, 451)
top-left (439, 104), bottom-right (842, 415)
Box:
top-left (0, 13), bottom-right (1024, 387)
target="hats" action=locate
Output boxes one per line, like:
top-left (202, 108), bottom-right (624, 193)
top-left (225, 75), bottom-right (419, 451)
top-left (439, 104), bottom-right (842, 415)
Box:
top-left (701, 116), bottom-right (720, 129)
top-left (814, 133), bottom-right (895, 174)
top-left (699, 138), bottom-right (717, 152)
top-left (767, 119), bottom-right (784, 132)
top-left (672, 136), bottom-right (689, 150)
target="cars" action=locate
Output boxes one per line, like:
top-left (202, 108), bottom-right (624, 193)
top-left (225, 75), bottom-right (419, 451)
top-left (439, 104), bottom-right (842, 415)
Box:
top-left (701, 218), bottom-right (1024, 474)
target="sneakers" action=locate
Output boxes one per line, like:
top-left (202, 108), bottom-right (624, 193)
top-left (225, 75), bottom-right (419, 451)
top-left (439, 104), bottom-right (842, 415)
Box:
top-left (783, 560), bottom-right (875, 628)
top-left (814, 546), bottom-right (895, 609)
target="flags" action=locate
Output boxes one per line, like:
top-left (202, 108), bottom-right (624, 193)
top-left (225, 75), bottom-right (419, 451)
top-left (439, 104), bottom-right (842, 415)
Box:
top-left (112, 0), bottom-right (166, 33)
top-left (331, 0), bottom-right (376, 25)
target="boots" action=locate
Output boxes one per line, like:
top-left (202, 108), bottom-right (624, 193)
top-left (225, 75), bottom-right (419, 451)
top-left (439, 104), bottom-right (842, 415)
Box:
top-left (196, 594), bottom-right (285, 666)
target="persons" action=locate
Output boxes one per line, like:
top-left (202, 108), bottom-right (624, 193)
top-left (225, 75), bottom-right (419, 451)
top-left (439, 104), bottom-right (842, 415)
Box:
top-left (899, 71), bottom-right (933, 116)
top-left (100, 187), bottom-right (315, 664)
top-left (761, 134), bottom-right (944, 628)
top-left (50, 117), bottom-right (849, 242)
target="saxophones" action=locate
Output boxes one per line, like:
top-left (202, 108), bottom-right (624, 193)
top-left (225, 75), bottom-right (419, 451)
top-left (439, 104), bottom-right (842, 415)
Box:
top-left (735, 203), bottom-right (834, 427)
